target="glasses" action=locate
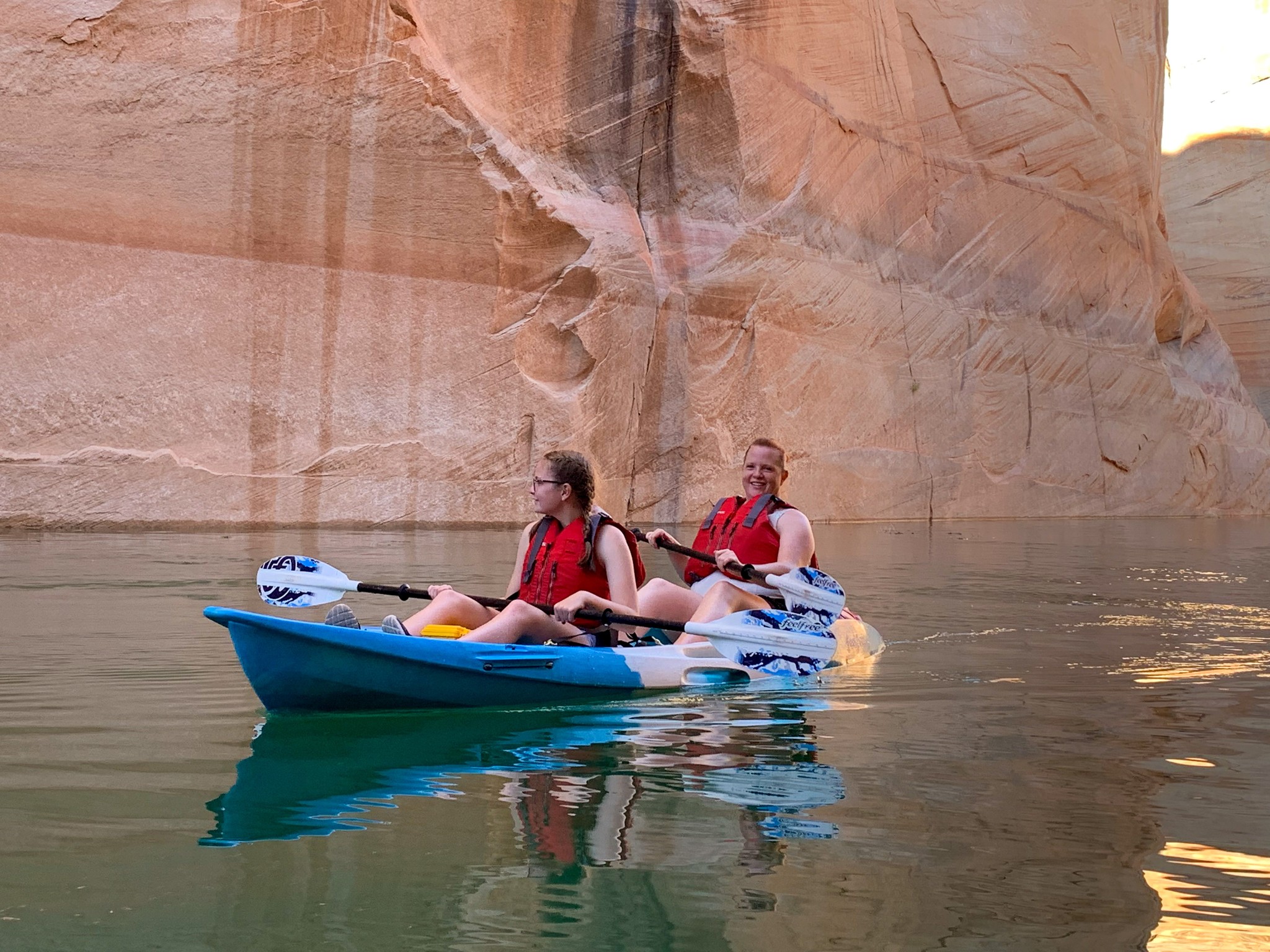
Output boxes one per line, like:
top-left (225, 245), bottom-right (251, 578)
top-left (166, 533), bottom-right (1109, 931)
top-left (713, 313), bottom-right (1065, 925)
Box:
top-left (533, 478), bottom-right (565, 491)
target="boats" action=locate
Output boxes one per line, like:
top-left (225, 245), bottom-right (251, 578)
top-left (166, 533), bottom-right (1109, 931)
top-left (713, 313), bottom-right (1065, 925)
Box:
top-left (201, 560), bottom-right (871, 713)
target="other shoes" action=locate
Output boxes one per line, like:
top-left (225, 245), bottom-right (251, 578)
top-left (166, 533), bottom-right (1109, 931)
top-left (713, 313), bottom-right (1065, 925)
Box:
top-left (382, 614), bottom-right (412, 636)
top-left (324, 603), bottom-right (361, 629)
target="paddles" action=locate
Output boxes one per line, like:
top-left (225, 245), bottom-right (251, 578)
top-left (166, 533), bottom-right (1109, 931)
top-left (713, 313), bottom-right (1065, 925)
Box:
top-left (255, 554), bottom-right (837, 679)
top-left (631, 525), bottom-right (846, 627)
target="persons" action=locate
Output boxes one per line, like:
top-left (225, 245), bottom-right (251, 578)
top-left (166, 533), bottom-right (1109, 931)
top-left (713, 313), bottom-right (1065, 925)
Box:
top-left (616, 437), bottom-right (820, 649)
top-left (323, 450), bottom-right (639, 649)
